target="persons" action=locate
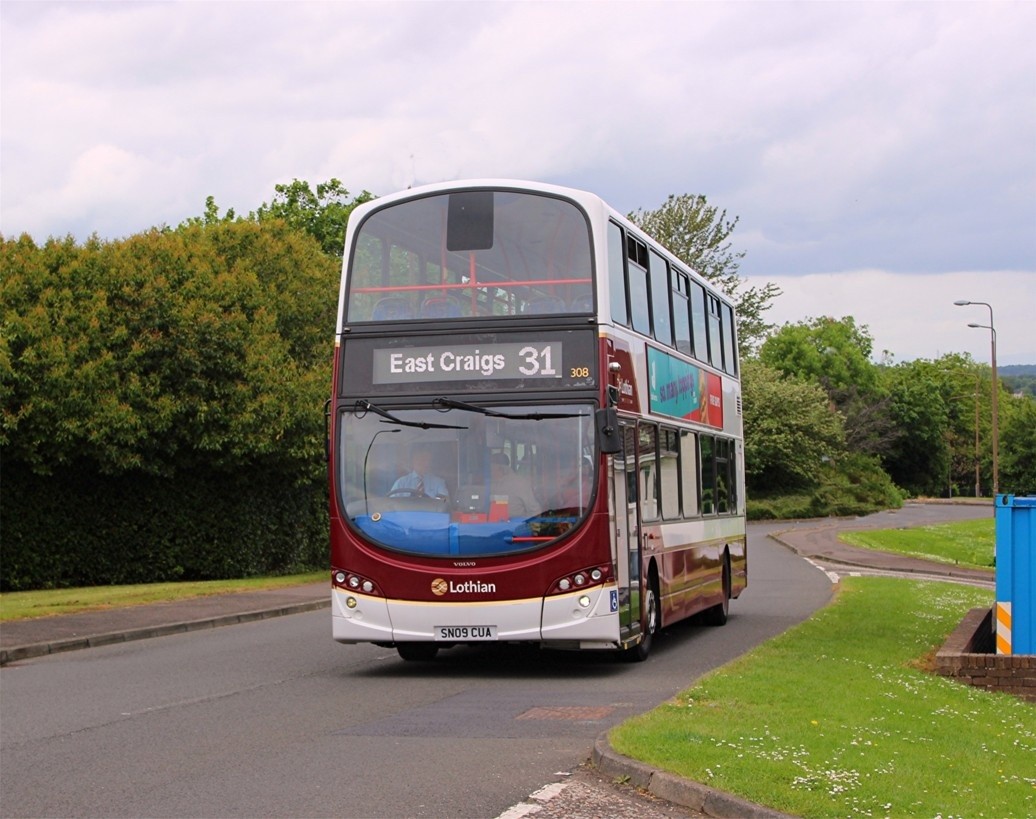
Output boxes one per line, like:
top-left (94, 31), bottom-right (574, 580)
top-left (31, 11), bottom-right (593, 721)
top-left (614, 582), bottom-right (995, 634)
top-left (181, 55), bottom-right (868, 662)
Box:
top-left (544, 458), bottom-right (588, 515)
top-left (486, 452), bottom-right (541, 536)
top-left (389, 449), bottom-right (449, 503)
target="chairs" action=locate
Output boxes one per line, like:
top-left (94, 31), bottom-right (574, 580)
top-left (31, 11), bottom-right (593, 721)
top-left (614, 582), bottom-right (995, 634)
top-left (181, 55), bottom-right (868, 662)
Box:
top-left (372, 297), bottom-right (415, 321)
top-left (419, 297), bottom-right (464, 317)
top-left (523, 295), bottom-right (566, 312)
top-left (570, 293), bottom-right (593, 312)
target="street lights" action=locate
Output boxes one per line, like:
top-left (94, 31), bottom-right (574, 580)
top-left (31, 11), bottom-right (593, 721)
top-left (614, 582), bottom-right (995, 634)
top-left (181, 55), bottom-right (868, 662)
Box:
top-left (953, 300), bottom-right (999, 517)
top-left (951, 370), bottom-right (980, 499)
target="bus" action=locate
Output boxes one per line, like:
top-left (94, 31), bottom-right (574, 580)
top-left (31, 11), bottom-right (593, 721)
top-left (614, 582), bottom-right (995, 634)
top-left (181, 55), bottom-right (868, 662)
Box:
top-left (324, 178), bottom-right (748, 664)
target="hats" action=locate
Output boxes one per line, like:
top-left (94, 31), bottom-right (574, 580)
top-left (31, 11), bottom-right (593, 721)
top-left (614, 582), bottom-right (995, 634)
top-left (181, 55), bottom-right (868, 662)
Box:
top-left (491, 453), bottom-right (510, 465)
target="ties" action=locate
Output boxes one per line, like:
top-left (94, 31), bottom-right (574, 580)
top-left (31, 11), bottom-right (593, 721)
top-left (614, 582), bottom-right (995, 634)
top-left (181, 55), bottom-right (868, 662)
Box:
top-left (416, 478), bottom-right (425, 492)
